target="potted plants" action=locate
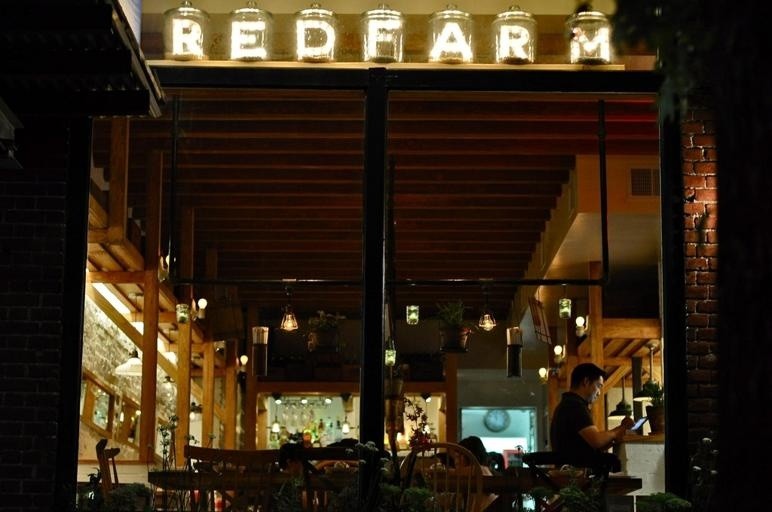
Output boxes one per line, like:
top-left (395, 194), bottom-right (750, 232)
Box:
top-left (642, 383), bottom-right (666, 435)
top-left (436, 299), bottom-right (472, 349)
top-left (384, 338), bottom-right (410, 395)
top-left (305, 310), bottom-right (346, 349)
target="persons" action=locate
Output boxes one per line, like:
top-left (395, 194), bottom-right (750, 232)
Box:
top-left (549, 363), bottom-right (636, 473)
top-left (431, 436), bottom-right (507, 512)
top-left (268, 443), bottom-right (306, 511)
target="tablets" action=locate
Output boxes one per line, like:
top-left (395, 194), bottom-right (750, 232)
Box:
top-left (630, 416), bottom-right (648, 431)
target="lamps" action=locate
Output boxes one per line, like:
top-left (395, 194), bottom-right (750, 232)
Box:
top-left (185, 356), bottom-right (201, 420)
top-left (281, 303), bottom-right (298, 333)
top-left (478, 291), bottom-right (496, 331)
top-left (407, 283), bottom-right (420, 325)
top-left (385, 319), bottom-right (396, 367)
top-left (558, 284), bottom-right (571, 319)
top-left (250, 325), bottom-right (269, 375)
top-left (238, 354), bottom-right (249, 372)
top-left (575, 314), bottom-right (590, 337)
top-left (158, 231), bottom-right (173, 284)
top-left (633, 341), bottom-right (663, 402)
top-left (174, 298), bottom-right (191, 322)
top-left (538, 366), bottom-right (551, 383)
top-left (271, 392), bottom-right (354, 434)
top-left (116, 298), bottom-right (143, 375)
top-left (553, 344), bottom-right (564, 363)
top-left (193, 298), bottom-right (208, 320)
top-left (608, 377), bottom-right (633, 421)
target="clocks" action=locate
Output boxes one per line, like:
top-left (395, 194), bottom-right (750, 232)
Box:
top-left (484, 408), bottom-right (510, 432)
